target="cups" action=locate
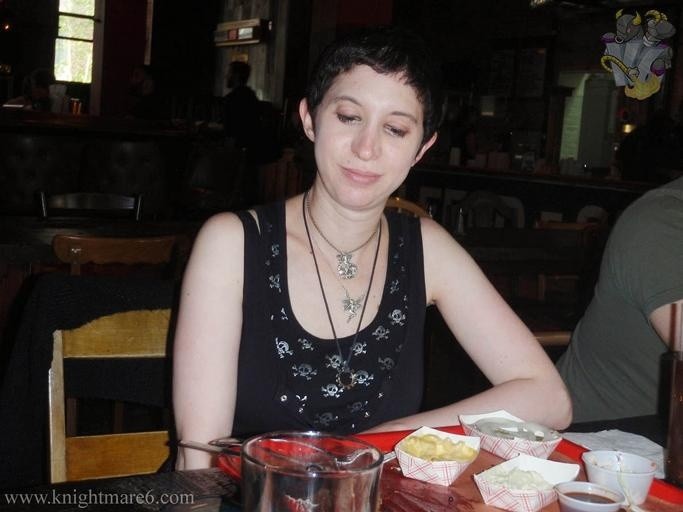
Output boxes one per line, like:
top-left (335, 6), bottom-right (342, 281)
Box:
top-left (241, 432), bottom-right (382, 512)
top-left (69, 98), bottom-right (80, 115)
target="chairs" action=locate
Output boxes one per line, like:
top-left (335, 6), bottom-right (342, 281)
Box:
top-left (1, 191), bottom-right (612, 485)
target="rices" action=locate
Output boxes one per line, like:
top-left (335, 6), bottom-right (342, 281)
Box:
top-left (486, 467), bottom-right (552, 492)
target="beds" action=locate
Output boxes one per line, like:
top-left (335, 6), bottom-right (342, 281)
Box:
top-left (0, 467), bottom-right (238, 512)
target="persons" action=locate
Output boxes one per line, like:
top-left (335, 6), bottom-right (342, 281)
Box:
top-left (1, 67), bottom-right (56, 111)
top-left (553, 173), bottom-right (682, 424)
top-left (220, 56), bottom-right (258, 101)
top-left (152, 28), bottom-right (574, 476)
top-left (115, 63), bottom-right (170, 121)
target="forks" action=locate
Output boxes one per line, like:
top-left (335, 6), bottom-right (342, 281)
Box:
top-left (209, 436), bottom-right (367, 465)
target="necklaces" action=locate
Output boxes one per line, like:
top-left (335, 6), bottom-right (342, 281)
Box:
top-left (306, 199), bottom-right (380, 324)
top-left (298, 193), bottom-right (385, 395)
top-left (308, 205), bottom-right (379, 279)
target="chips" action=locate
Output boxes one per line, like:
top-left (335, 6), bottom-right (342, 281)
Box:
top-left (402, 434), bottom-right (475, 462)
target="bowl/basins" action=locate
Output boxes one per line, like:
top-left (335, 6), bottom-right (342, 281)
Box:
top-left (554, 451), bottom-right (659, 512)
